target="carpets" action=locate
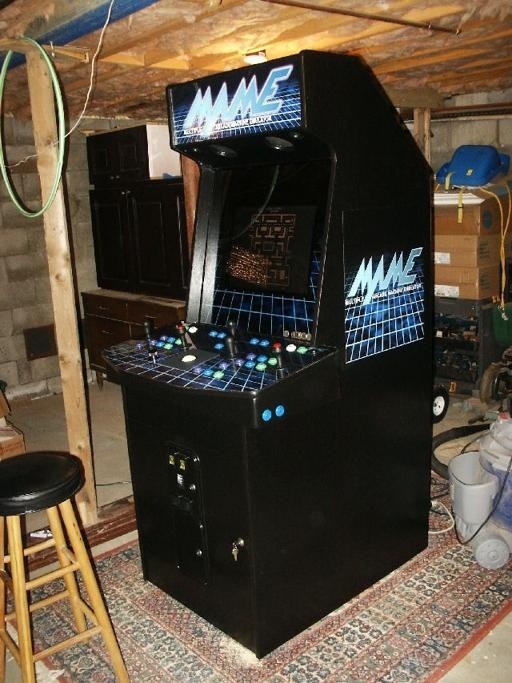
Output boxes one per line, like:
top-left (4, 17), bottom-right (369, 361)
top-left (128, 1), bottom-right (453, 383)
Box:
top-left (0, 452), bottom-right (511, 683)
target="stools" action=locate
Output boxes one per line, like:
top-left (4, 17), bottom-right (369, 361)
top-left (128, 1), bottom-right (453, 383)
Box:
top-left (0, 450), bottom-right (131, 683)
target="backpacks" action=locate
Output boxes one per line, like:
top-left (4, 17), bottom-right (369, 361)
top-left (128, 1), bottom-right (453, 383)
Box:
top-left (438, 144), bottom-right (511, 224)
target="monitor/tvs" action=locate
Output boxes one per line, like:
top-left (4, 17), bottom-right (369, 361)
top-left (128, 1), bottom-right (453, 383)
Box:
top-left (216, 197), bottom-right (316, 303)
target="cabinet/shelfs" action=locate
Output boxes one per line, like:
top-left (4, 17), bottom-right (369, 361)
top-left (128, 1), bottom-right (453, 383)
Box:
top-left (79, 124), bottom-right (200, 395)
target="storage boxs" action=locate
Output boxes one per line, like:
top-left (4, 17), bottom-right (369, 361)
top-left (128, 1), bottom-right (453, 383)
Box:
top-left (433, 184), bottom-right (512, 301)
